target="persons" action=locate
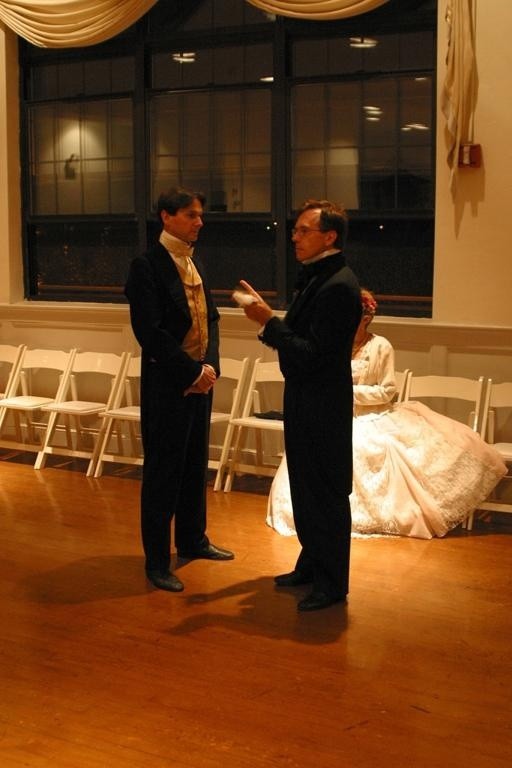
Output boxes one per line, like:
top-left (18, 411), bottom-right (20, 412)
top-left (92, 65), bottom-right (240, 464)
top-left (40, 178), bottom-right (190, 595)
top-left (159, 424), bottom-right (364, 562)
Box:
top-left (222, 197), bottom-right (362, 615)
top-left (263, 287), bottom-right (509, 541)
top-left (119, 182), bottom-right (235, 596)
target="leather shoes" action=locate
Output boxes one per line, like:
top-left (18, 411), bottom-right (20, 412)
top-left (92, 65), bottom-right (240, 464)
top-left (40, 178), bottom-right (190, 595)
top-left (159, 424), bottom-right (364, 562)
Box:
top-left (147, 569), bottom-right (184, 592)
top-left (178, 542), bottom-right (234, 559)
top-left (272, 571), bottom-right (304, 586)
top-left (297, 597), bottom-right (320, 612)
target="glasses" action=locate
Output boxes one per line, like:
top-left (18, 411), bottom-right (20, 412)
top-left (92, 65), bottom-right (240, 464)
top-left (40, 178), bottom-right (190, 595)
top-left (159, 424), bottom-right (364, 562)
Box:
top-left (291, 229), bottom-right (322, 235)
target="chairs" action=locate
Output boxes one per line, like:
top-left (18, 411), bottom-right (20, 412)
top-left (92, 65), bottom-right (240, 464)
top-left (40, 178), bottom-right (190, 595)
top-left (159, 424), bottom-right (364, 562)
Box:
top-left (467, 378), bottom-right (511, 530)
top-left (0, 345), bottom-right (73, 470)
top-left (40, 347), bottom-right (127, 477)
top-left (391, 368), bottom-right (410, 402)
top-left (94, 352), bottom-right (144, 478)
top-left (223, 357), bottom-right (287, 493)
top-left (404, 372), bottom-right (484, 531)
top-left (1, 344), bottom-right (27, 460)
top-left (207, 356), bottom-right (250, 492)
